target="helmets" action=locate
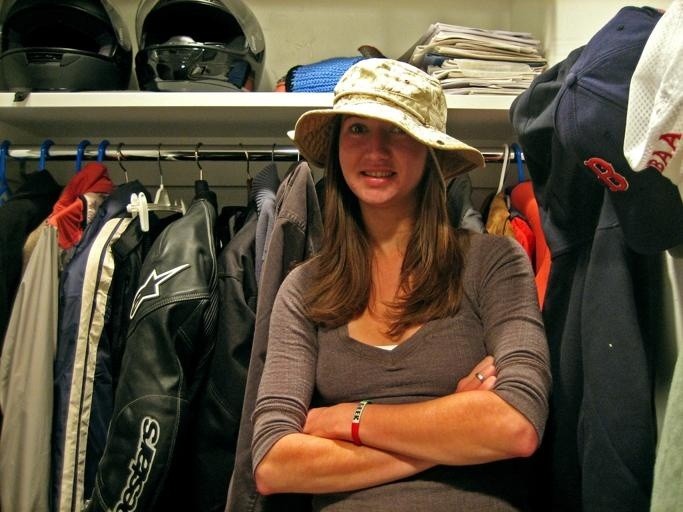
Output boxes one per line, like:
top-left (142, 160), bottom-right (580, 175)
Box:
top-left (133, 1), bottom-right (267, 91)
top-left (0, 0), bottom-right (135, 91)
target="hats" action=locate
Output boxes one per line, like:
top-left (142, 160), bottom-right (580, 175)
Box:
top-left (510, 3), bottom-right (683, 258)
top-left (294, 57), bottom-right (486, 181)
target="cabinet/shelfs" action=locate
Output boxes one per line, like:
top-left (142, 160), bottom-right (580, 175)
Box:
top-left (2, 1), bottom-right (563, 134)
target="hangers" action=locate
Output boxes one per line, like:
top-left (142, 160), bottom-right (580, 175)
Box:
top-left (0, 138), bottom-right (526, 234)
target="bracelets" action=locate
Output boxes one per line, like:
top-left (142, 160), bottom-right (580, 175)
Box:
top-left (351, 400), bottom-right (373, 446)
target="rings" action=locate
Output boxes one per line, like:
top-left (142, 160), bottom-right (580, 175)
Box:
top-left (474, 372), bottom-right (486, 382)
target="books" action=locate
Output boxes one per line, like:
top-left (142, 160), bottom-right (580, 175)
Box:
top-left (398, 20), bottom-right (549, 94)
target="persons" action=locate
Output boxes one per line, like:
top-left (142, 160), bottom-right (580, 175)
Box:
top-left (248, 56), bottom-right (554, 509)
